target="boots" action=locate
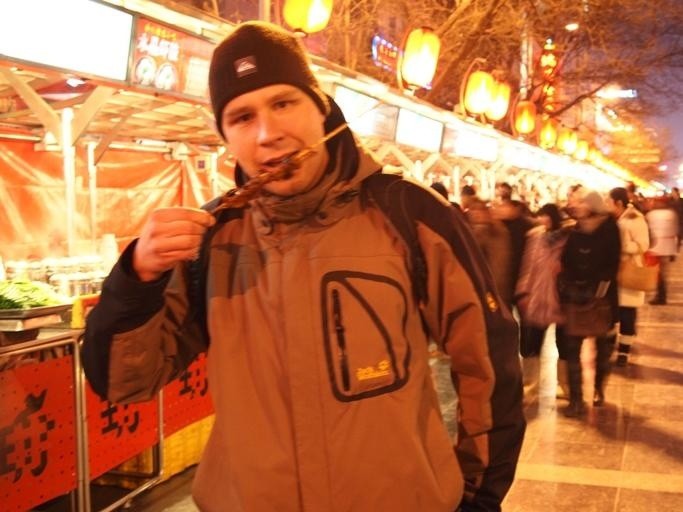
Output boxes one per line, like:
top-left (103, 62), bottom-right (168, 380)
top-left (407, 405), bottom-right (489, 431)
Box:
top-left (608, 327), bottom-right (635, 366)
top-left (593, 361), bottom-right (609, 404)
top-left (557, 359), bottom-right (585, 417)
top-left (648, 282), bottom-right (666, 304)
top-left (523, 358), bottom-right (540, 413)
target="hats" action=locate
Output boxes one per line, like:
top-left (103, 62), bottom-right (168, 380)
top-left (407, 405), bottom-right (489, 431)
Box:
top-left (209, 20), bottom-right (331, 141)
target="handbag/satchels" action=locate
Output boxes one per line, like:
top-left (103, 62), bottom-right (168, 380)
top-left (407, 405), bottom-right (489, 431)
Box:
top-left (566, 298), bottom-right (613, 337)
top-left (616, 256), bottom-right (660, 290)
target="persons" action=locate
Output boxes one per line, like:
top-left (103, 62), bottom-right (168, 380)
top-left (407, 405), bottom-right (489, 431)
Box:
top-left (435, 182), bottom-right (583, 406)
top-left (79, 18), bottom-right (525, 512)
top-left (564, 190), bottom-right (622, 415)
top-left (608, 181), bottom-right (682, 365)
